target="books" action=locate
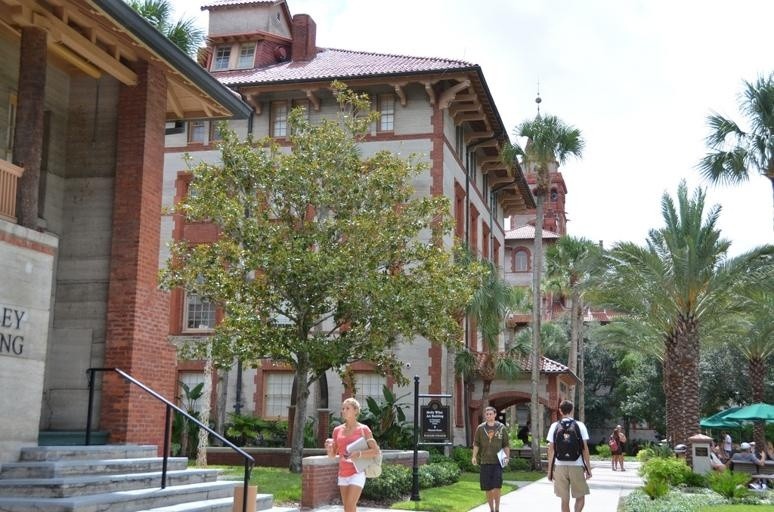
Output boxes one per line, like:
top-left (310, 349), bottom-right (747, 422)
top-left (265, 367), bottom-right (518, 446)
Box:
top-left (496, 447), bottom-right (509, 468)
top-left (346, 436), bottom-right (374, 473)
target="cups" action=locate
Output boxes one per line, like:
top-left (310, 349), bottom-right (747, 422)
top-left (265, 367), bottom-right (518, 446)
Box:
top-left (327, 439), bottom-right (333, 446)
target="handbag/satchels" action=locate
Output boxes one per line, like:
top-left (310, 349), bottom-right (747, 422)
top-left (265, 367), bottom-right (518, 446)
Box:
top-left (361, 424), bottom-right (383, 479)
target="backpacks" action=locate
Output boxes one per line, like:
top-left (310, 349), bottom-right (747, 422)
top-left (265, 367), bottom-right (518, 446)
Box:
top-left (608, 439), bottom-right (618, 452)
top-left (552, 418), bottom-right (585, 461)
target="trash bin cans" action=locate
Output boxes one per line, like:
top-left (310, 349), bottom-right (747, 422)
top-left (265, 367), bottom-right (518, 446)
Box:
top-left (687, 434), bottom-right (714, 476)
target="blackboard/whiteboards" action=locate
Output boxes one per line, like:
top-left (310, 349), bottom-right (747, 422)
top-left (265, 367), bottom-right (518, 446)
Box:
top-left (420, 400), bottom-right (450, 440)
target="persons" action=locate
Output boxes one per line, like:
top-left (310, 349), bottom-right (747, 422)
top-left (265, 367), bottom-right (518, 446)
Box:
top-left (708, 429), bottom-right (774, 482)
top-left (545, 399), bottom-right (592, 512)
top-left (323, 395), bottom-right (379, 512)
top-left (608, 428), bottom-right (621, 471)
top-left (471, 405), bottom-right (510, 512)
top-left (516, 421), bottom-right (531, 447)
top-left (616, 424), bottom-right (626, 471)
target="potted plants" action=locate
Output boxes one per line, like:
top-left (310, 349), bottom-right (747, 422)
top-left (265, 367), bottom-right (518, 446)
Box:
top-left (540, 457), bottom-right (548, 471)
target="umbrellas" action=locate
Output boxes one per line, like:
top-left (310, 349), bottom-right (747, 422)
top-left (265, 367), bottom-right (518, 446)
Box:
top-left (699, 403), bottom-right (765, 447)
top-left (721, 401), bottom-right (773, 452)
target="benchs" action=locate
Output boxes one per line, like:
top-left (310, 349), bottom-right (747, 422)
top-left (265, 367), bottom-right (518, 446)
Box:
top-left (509, 449), bottom-right (532, 459)
top-left (731, 460), bottom-right (774, 489)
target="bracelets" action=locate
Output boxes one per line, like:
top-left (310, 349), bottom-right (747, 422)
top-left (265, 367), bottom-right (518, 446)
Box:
top-left (358, 450), bottom-right (363, 459)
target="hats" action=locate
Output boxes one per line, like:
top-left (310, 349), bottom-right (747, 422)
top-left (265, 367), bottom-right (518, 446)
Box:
top-left (739, 442), bottom-right (751, 450)
